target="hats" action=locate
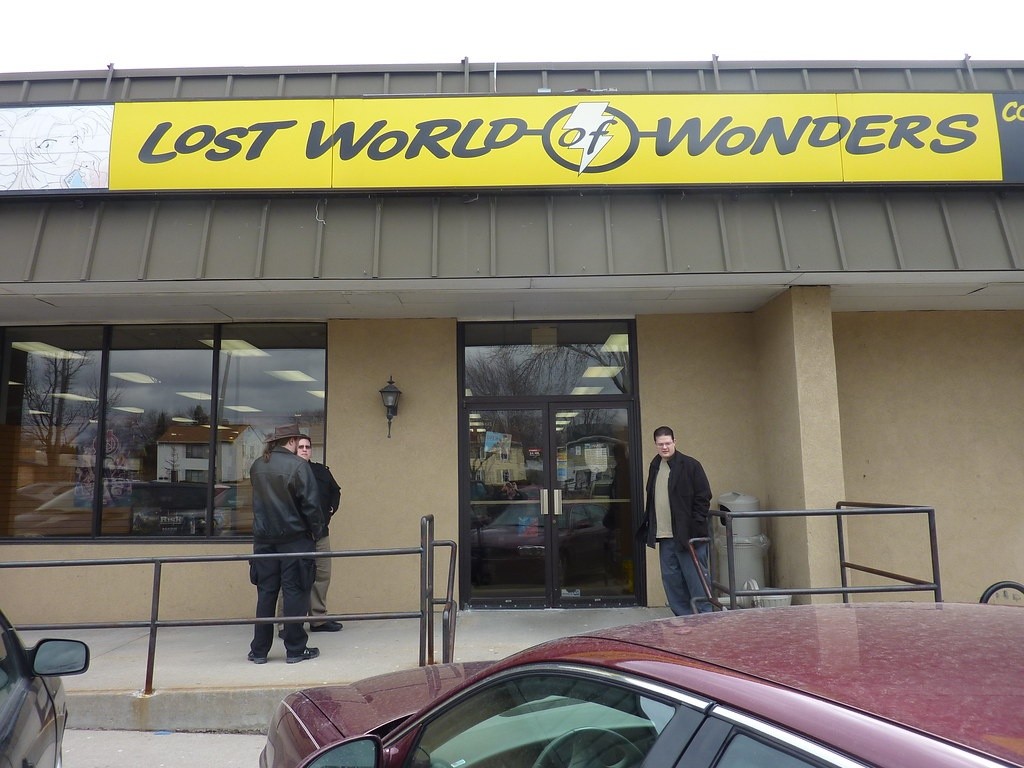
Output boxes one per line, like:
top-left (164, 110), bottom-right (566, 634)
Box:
top-left (264, 423), bottom-right (302, 442)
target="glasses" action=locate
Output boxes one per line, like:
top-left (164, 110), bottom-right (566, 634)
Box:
top-left (299, 445), bottom-right (311, 450)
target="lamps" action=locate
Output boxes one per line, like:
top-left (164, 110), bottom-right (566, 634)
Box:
top-left (378, 375), bottom-right (403, 436)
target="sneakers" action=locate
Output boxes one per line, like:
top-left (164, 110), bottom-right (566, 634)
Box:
top-left (248, 650), bottom-right (266, 664)
top-left (286, 647), bottom-right (320, 663)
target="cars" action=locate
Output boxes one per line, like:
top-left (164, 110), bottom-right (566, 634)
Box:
top-left (590, 479), bottom-right (615, 497)
top-left (471, 502), bottom-right (612, 583)
top-left (16, 482), bottom-right (78, 514)
top-left (255, 604), bottom-right (1024, 768)
top-left (11, 477), bottom-right (148, 536)
top-left (134, 484), bottom-right (253, 536)
top-left (0, 609), bottom-right (91, 768)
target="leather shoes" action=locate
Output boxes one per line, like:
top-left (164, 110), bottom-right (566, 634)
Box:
top-left (310, 621), bottom-right (342, 631)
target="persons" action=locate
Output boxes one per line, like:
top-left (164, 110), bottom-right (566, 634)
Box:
top-left (248, 423), bottom-right (325, 664)
top-left (487, 482), bottom-right (528, 518)
top-left (634, 426), bottom-right (713, 616)
top-left (601, 444), bottom-right (634, 595)
top-left (277, 434), bottom-right (341, 640)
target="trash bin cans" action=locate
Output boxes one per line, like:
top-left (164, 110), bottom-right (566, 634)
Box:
top-left (716, 490), bottom-right (765, 598)
